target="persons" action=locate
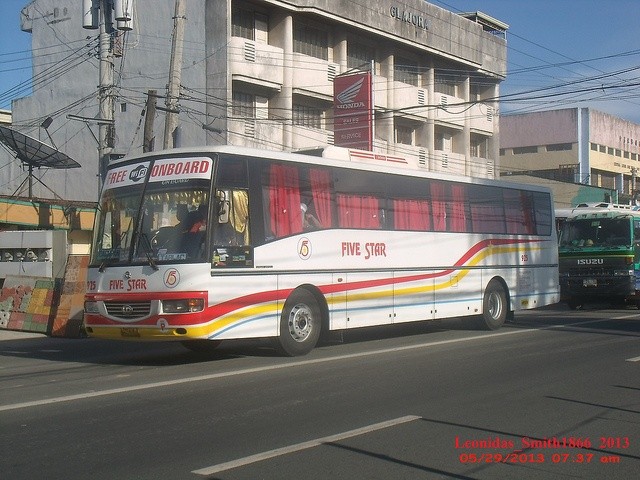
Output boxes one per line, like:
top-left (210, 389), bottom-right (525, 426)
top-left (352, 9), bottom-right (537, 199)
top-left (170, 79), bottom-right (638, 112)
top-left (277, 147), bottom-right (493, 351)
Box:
top-left (298, 202), bottom-right (322, 230)
top-left (174, 204), bottom-right (190, 228)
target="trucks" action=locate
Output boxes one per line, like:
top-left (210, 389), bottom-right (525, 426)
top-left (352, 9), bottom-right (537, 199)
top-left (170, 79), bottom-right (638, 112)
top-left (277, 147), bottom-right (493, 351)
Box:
top-left (559, 213), bottom-right (640, 296)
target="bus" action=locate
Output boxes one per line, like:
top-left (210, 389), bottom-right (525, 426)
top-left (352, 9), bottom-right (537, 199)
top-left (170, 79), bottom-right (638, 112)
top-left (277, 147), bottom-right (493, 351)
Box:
top-left (84, 146), bottom-right (561, 357)
